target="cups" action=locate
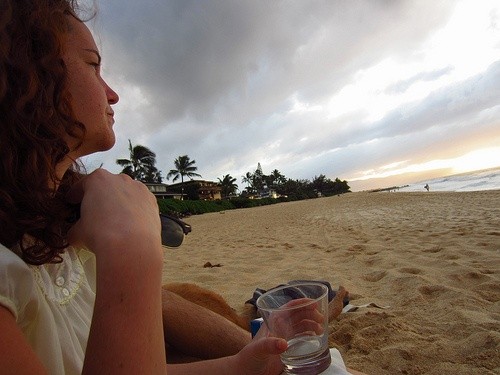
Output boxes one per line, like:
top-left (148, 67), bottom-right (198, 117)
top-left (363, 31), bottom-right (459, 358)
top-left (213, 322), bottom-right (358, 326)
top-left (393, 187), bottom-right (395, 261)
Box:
top-left (256, 282), bottom-right (331, 375)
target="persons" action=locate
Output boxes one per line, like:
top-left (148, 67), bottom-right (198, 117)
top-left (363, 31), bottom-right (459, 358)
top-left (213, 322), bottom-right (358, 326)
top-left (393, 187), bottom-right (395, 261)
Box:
top-left (0, 0), bottom-right (325, 375)
top-left (425, 183), bottom-right (430, 191)
top-left (160, 281), bottom-right (350, 357)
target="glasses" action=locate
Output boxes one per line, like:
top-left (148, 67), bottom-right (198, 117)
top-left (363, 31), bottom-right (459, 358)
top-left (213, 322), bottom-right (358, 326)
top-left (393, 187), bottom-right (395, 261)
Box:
top-left (160, 211), bottom-right (193, 251)
